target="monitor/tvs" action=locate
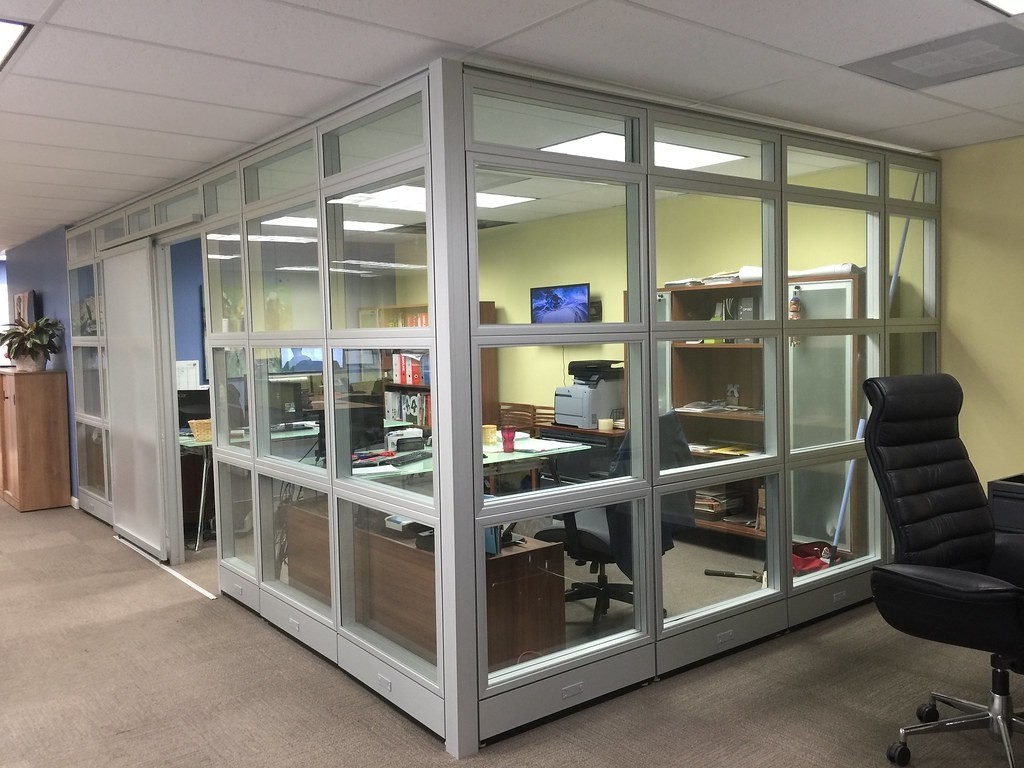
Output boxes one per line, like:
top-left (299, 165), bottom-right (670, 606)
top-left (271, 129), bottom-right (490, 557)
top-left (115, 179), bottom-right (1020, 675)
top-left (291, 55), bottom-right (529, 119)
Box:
top-left (318, 407), bottom-right (384, 458)
top-left (268, 381), bottom-right (303, 425)
top-left (176, 390), bottom-right (211, 428)
top-left (280, 349), bottom-right (343, 373)
top-left (530, 282), bottom-right (590, 324)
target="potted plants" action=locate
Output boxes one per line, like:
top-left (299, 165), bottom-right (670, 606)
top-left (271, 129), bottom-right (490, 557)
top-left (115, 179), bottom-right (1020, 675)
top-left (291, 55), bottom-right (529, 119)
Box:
top-left (0, 317), bottom-right (65, 373)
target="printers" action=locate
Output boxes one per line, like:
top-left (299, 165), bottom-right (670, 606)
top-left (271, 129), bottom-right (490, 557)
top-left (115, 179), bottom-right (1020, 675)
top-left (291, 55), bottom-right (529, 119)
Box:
top-left (554, 361), bottom-right (625, 430)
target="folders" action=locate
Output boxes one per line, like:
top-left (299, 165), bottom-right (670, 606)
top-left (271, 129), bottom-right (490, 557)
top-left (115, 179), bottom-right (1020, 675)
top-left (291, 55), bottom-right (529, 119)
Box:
top-left (391, 354), bottom-right (429, 386)
top-left (385, 392), bottom-right (400, 421)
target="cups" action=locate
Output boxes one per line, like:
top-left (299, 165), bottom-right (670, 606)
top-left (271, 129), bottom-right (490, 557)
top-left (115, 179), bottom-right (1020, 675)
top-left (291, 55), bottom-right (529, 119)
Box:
top-left (501, 426), bottom-right (516, 452)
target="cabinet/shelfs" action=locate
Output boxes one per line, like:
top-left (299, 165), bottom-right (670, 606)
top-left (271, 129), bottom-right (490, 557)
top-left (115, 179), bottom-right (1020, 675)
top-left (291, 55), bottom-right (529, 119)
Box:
top-left (378, 301), bottom-right (500, 432)
top-left (0, 367), bottom-right (72, 512)
top-left (622, 272), bottom-right (866, 561)
top-left (987, 473), bottom-right (1024, 536)
top-left (285, 496), bottom-right (566, 675)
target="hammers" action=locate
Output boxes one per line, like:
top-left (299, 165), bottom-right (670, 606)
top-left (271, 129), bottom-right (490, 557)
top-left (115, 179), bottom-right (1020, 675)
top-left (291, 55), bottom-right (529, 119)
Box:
top-left (704, 568), bottom-right (763, 582)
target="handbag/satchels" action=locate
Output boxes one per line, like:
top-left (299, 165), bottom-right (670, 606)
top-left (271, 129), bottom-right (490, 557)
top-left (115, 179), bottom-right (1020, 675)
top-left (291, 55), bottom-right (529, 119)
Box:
top-left (790, 540), bottom-right (843, 577)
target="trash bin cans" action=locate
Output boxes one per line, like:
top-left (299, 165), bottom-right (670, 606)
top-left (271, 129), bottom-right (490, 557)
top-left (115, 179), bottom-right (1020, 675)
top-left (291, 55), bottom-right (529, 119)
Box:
top-left (986, 471), bottom-right (1024, 534)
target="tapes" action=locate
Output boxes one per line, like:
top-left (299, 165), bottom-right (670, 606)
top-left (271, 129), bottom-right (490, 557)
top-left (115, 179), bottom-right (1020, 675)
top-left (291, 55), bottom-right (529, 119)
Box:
top-left (599, 418), bottom-right (614, 432)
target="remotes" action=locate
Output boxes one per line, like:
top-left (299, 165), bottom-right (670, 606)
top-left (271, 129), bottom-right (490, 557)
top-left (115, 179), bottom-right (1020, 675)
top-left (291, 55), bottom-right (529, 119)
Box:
top-left (352, 461), bottom-right (377, 468)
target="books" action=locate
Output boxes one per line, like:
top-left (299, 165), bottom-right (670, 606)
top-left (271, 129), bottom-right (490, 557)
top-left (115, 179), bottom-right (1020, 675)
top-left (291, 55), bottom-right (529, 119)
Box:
top-left (723, 298), bottom-right (734, 343)
top-left (675, 405), bottom-right (765, 455)
top-left (664, 271), bottom-right (739, 288)
top-left (383, 312), bottom-right (432, 426)
top-left (759, 488), bottom-right (766, 532)
top-left (694, 488), bottom-right (746, 521)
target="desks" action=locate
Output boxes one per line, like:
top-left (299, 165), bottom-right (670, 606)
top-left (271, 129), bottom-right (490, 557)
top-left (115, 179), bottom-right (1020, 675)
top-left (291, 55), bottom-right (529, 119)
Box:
top-left (275, 430), bottom-right (593, 580)
top-left (179, 419), bottom-right (414, 552)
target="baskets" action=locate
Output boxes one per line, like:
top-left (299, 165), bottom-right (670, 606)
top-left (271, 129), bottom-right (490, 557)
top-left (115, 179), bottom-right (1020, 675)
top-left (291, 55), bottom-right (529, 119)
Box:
top-left (188, 419), bottom-right (213, 442)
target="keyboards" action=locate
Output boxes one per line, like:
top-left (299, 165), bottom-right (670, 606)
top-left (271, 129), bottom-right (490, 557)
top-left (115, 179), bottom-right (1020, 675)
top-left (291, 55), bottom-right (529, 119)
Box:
top-left (386, 451), bottom-right (433, 466)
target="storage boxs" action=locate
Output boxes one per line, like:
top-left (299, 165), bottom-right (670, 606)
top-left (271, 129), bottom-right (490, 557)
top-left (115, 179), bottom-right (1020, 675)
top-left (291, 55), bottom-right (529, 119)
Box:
top-left (392, 354), bottom-right (430, 386)
top-left (384, 390), bottom-right (402, 421)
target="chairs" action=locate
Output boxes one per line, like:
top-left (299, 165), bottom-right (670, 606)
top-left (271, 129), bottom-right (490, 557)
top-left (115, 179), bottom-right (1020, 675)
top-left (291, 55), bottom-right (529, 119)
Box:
top-left (489, 402), bottom-right (555, 495)
top-left (534, 410), bottom-right (696, 642)
top-left (862, 374), bottom-right (1024, 768)
